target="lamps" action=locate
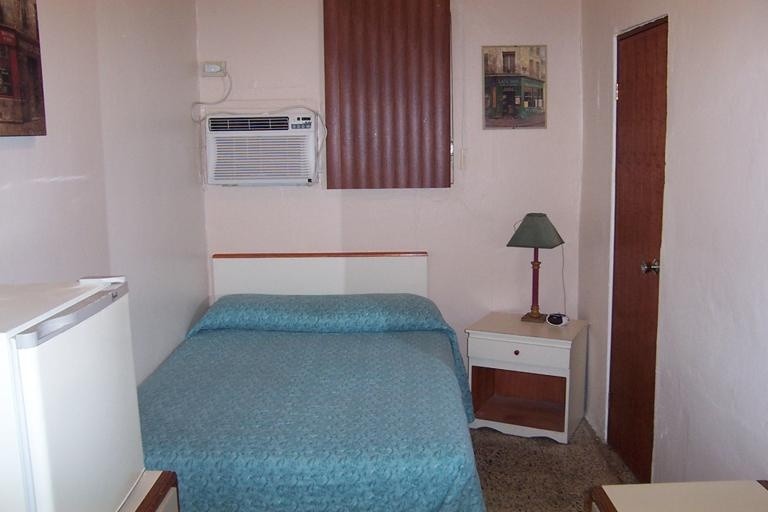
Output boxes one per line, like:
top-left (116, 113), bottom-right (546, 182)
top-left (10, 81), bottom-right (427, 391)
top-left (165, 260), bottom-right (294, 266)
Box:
top-left (506, 212), bottom-right (564, 323)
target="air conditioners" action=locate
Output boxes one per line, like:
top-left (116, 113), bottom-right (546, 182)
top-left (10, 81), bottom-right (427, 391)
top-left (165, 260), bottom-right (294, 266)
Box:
top-left (206, 114), bottom-right (318, 186)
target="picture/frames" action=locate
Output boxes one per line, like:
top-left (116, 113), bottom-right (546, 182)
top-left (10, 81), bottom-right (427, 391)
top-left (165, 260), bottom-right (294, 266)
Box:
top-left (481, 45), bottom-right (548, 130)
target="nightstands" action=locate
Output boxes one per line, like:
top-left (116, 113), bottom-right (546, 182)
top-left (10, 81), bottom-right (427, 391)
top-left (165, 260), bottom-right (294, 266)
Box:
top-left (465, 312), bottom-right (588, 444)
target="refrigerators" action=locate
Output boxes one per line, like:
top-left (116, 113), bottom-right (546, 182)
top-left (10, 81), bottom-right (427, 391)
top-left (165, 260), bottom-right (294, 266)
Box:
top-left (1, 277), bottom-right (145, 512)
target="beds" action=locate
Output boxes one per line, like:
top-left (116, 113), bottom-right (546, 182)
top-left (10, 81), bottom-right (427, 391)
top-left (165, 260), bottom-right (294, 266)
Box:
top-left (139, 250), bottom-right (483, 511)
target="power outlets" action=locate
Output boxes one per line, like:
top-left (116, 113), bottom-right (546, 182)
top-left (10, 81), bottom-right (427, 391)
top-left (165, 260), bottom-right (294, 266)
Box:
top-left (204, 61), bottom-right (227, 78)
